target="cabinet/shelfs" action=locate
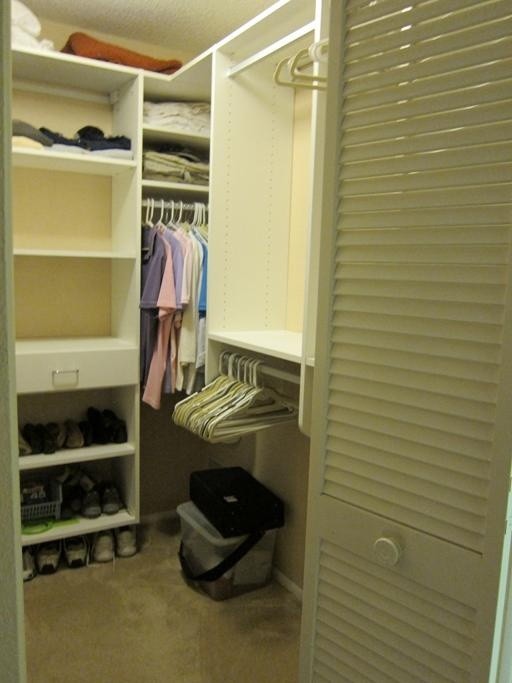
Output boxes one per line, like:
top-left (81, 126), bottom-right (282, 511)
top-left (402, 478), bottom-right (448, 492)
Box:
top-left (12, 50), bottom-right (143, 550)
top-left (142, 75), bottom-right (215, 190)
top-left (204, 1), bottom-right (326, 439)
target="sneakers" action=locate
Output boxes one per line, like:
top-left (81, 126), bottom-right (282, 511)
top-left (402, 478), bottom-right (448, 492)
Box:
top-left (17, 407), bottom-right (126, 455)
top-left (59, 464), bottom-right (123, 521)
top-left (22, 526), bottom-right (138, 582)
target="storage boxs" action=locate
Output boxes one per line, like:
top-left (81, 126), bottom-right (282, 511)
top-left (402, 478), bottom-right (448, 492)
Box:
top-left (176, 503), bottom-right (277, 600)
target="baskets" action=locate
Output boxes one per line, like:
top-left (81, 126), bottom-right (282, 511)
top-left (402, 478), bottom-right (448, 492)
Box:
top-left (22, 485), bottom-right (63, 522)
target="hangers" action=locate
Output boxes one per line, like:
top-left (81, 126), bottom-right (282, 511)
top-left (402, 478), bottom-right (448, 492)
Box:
top-left (144, 197), bottom-right (209, 224)
top-left (172, 351), bottom-right (301, 444)
top-left (273, 34), bottom-right (334, 92)
